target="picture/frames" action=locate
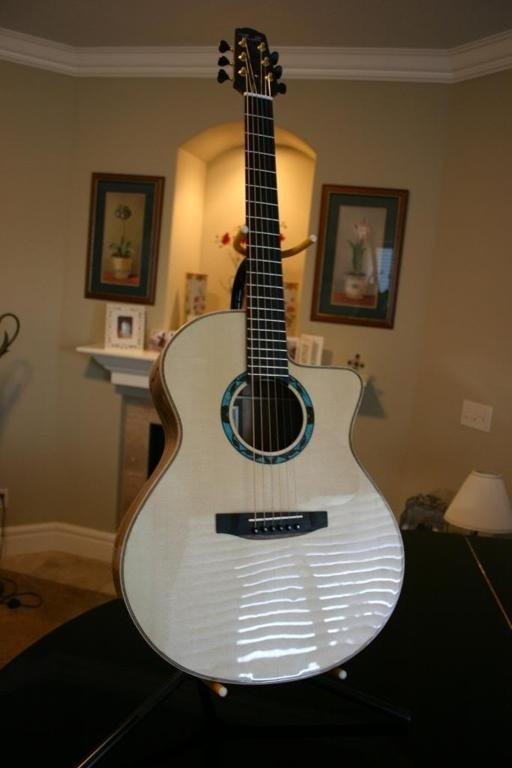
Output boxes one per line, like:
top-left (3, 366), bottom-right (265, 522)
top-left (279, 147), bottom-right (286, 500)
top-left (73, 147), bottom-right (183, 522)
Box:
top-left (310, 182), bottom-right (408, 328)
top-left (83, 169), bottom-right (164, 309)
top-left (106, 304), bottom-right (145, 352)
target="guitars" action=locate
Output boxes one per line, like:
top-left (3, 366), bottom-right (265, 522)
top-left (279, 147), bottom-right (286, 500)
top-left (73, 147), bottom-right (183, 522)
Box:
top-left (111, 29), bottom-right (405, 684)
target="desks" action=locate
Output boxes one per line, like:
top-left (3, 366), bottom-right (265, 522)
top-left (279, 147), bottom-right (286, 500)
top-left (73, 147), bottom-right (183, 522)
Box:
top-left (1, 534), bottom-right (512, 768)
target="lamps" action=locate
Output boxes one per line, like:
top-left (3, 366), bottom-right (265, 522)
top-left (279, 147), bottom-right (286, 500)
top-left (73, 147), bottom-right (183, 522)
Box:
top-left (444, 467), bottom-right (511, 536)
